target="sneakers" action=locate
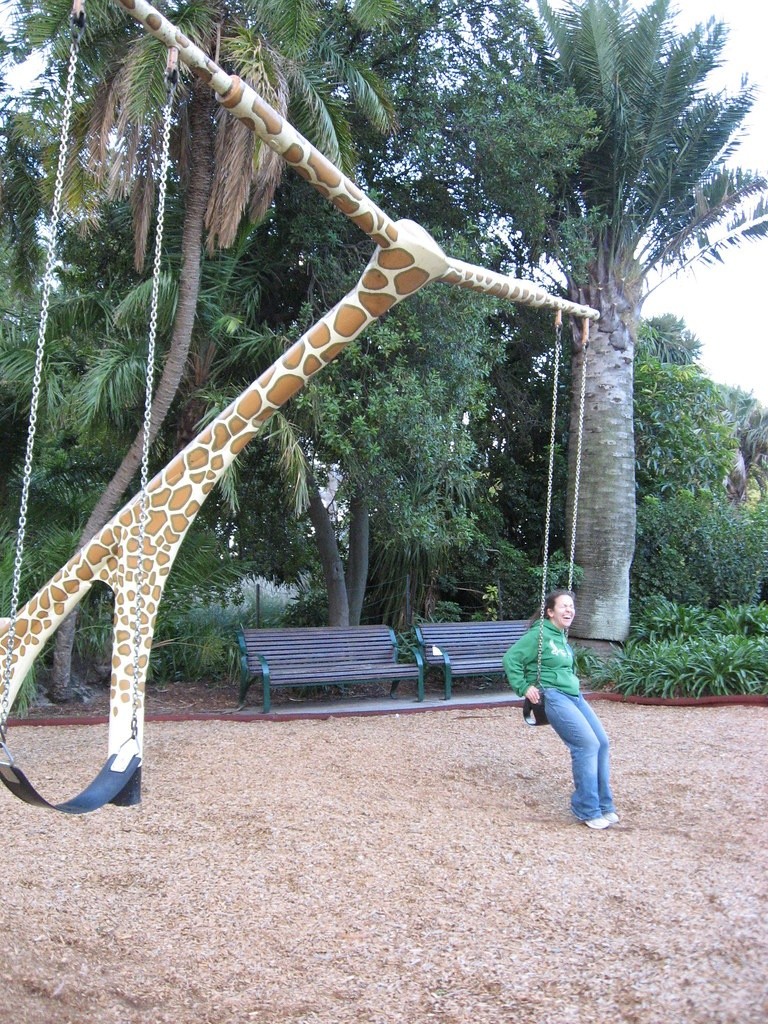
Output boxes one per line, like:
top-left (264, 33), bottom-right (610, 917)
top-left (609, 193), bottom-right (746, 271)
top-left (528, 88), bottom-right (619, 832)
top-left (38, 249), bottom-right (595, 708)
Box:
top-left (572, 812), bottom-right (618, 829)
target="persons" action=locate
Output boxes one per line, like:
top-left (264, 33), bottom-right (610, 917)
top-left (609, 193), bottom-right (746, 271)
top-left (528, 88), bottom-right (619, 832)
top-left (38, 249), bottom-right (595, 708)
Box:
top-left (501, 591), bottom-right (621, 829)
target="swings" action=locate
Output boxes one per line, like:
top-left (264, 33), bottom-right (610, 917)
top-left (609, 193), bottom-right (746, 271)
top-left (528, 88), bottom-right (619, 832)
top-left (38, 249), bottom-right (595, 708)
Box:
top-left (0, 23), bottom-right (184, 819)
top-left (522, 309), bottom-right (592, 727)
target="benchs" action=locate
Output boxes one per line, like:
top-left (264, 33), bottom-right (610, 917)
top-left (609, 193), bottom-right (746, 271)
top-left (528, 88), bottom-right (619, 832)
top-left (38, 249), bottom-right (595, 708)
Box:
top-left (234, 625), bottom-right (424, 714)
top-left (411, 618), bottom-right (532, 700)
